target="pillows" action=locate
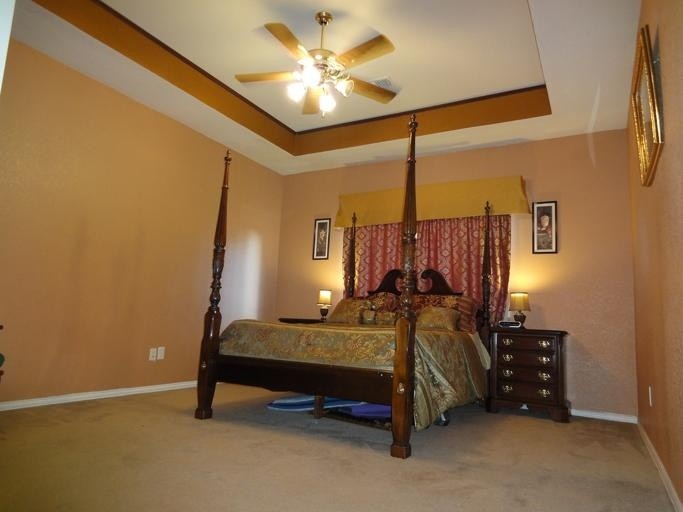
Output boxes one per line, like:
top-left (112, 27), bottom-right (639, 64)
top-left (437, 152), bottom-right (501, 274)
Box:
top-left (328, 292), bottom-right (477, 330)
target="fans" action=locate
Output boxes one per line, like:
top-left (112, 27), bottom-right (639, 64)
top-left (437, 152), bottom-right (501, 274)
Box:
top-left (235, 12), bottom-right (397, 113)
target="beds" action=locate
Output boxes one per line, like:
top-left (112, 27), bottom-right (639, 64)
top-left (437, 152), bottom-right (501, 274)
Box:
top-left (195, 113), bottom-right (491, 458)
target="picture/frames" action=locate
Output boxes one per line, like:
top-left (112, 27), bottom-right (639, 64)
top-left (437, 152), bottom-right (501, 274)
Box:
top-left (630, 23), bottom-right (664, 186)
top-left (532, 201), bottom-right (557, 254)
top-left (313, 218), bottom-right (330, 259)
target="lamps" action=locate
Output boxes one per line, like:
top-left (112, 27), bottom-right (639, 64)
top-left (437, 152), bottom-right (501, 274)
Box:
top-left (286, 57), bottom-right (354, 117)
top-left (509, 292), bottom-right (531, 329)
top-left (316, 290), bottom-right (332, 321)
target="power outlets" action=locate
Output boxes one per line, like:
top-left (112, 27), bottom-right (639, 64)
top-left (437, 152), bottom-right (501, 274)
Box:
top-left (149, 348), bottom-right (156, 361)
top-left (157, 347), bottom-right (165, 360)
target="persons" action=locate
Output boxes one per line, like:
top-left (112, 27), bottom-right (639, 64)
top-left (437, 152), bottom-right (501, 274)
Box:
top-left (537, 216), bottom-right (551, 239)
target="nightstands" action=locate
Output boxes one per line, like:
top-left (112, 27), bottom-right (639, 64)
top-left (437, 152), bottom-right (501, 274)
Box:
top-left (490, 327), bottom-right (569, 423)
top-left (278, 318), bottom-right (325, 323)
